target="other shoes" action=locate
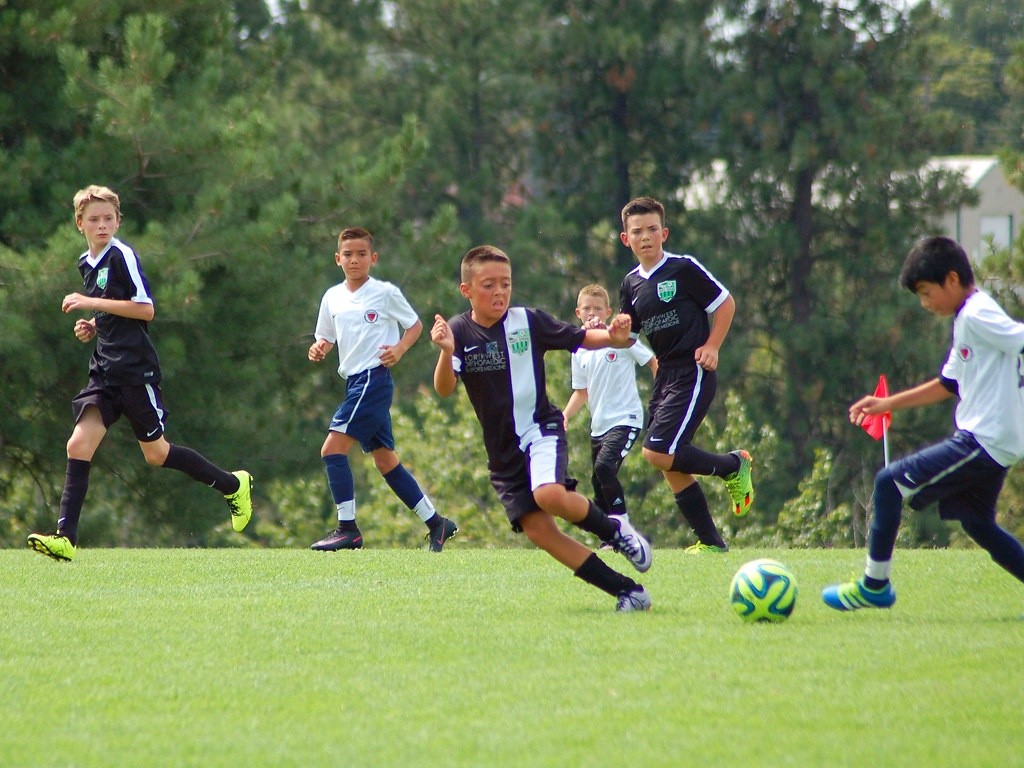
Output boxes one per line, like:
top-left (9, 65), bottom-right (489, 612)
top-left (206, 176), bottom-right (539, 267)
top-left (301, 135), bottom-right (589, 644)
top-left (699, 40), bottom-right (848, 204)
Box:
top-left (596, 542), bottom-right (613, 551)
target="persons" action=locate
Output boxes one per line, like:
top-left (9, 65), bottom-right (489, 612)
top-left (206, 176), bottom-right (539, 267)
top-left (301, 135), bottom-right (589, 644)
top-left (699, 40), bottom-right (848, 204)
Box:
top-left (561, 284), bottom-right (664, 556)
top-left (581, 196), bottom-right (755, 560)
top-left (307, 226), bottom-right (457, 551)
top-left (822, 235), bottom-right (1024, 628)
top-left (28, 183), bottom-right (255, 564)
top-left (431, 245), bottom-right (651, 615)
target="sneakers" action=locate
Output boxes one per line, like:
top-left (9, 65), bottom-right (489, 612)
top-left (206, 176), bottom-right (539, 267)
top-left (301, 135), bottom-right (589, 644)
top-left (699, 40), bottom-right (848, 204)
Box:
top-left (424, 517), bottom-right (457, 552)
top-left (27, 534), bottom-right (77, 563)
top-left (821, 572), bottom-right (896, 611)
top-left (600, 515), bottom-right (652, 573)
top-left (310, 529), bottom-right (363, 551)
top-left (223, 470), bottom-right (254, 533)
top-left (721, 450), bottom-right (754, 517)
top-left (683, 540), bottom-right (729, 555)
top-left (615, 584), bottom-right (651, 613)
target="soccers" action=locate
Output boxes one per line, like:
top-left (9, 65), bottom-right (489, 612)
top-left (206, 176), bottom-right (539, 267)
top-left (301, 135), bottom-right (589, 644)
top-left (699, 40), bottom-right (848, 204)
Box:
top-left (728, 558), bottom-right (799, 624)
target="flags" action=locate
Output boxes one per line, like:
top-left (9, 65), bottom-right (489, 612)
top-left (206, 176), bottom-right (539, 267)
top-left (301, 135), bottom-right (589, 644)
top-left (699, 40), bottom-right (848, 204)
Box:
top-left (859, 374), bottom-right (892, 444)
